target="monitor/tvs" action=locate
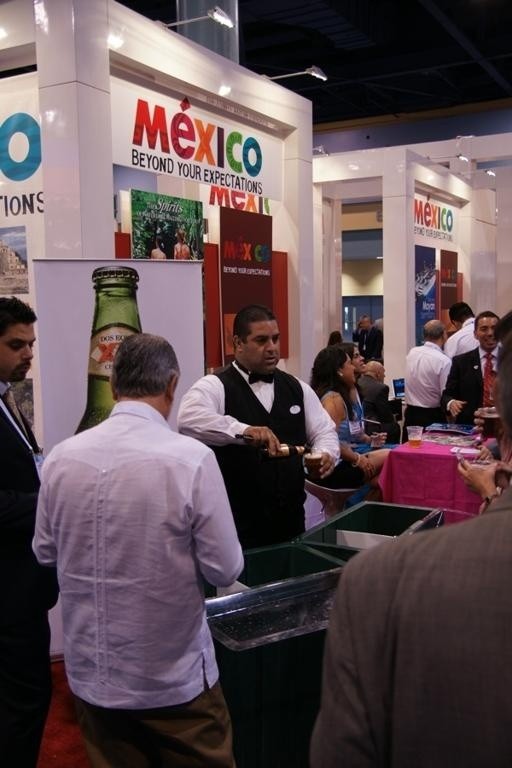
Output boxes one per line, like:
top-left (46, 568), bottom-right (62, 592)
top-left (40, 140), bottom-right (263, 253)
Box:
top-left (392, 378), bottom-right (405, 401)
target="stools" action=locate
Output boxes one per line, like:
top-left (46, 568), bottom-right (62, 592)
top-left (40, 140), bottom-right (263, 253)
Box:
top-left (304, 478), bottom-right (358, 521)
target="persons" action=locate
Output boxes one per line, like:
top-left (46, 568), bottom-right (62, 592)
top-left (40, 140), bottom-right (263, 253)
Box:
top-left (442, 311), bottom-right (502, 427)
top-left (172, 228), bottom-right (191, 260)
top-left (444, 303), bottom-right (482, 356)
top-left (0, 297), bottom-right (59, 767)
top-left (305, 337), bottom-right (511, 768)
top-left (457, 312), bottom-right (511, 512)
top-left (175, 302), bottom-right (340, 557)
top-left (31, 334), bottom-right (247, 766)
top-left (151, 235), bottom-right (167, 259)
top-left (401, 320), bottom-right (453, 442)
top-left (312, 344), bottom-right (401, 511)
top-left (353, 313), bottom-right (383, 359)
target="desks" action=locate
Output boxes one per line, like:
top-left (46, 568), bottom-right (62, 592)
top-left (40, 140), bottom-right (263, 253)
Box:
top-left (377, 431), bottom-right (485, 528)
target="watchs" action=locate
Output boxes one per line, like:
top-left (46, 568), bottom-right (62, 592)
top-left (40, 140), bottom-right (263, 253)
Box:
top-left (485, 495), bottom-right (498, 504)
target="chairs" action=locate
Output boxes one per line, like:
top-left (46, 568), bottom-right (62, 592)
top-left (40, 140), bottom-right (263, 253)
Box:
top-left (364, 419), bottom-right (383, 433)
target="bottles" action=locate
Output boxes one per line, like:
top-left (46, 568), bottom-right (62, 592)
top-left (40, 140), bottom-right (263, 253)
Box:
top-left (260, 443), bottom-right (307, 461)
top-left (70, 265), bottom-right (144, 438)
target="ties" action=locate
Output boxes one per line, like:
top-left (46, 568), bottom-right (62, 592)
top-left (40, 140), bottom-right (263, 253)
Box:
top-left (483, 352), bottom-right (495, 408)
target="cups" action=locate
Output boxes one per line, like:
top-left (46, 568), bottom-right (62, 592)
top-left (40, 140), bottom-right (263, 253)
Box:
top-left (447, 413), bottom-right (456, 428)
top-left (463, 460), bottom-right (496, 493)
top-left (304, 449), bottom-right (324, 483)
top-left (404, 425), bottom-right (424, 449)
top-left (478, 407), bottom-right (500, 439)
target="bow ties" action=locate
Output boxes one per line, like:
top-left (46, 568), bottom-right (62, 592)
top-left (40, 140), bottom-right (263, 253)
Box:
top-left (249, 369), bottom-right (275, 385)
